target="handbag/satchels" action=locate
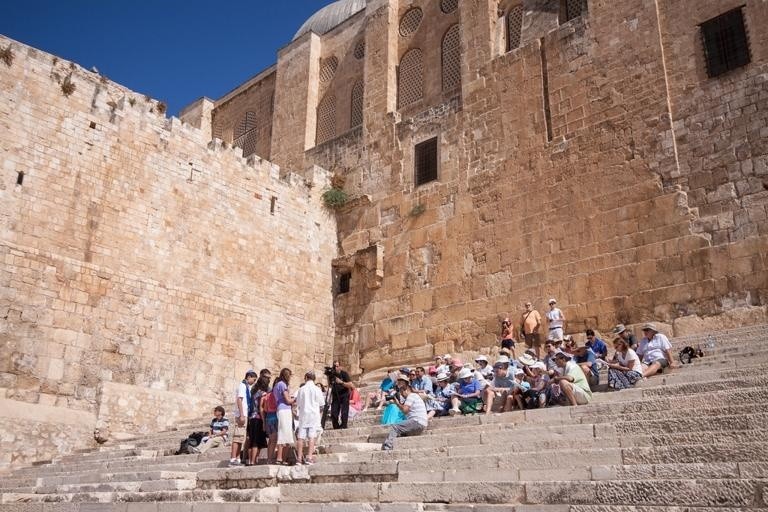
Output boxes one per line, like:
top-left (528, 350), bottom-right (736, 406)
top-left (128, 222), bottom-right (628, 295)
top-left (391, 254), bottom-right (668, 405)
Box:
top-left (522, 325), bottom-right (524, 335)
top-left (180, 432), bottom-right (209, 453)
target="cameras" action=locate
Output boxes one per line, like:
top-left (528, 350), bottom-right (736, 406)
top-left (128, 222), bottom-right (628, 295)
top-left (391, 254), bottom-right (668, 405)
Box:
top-left (502, 322), bottom-right (507, 328)
top-left (385, 392), bottom-right (401, 402)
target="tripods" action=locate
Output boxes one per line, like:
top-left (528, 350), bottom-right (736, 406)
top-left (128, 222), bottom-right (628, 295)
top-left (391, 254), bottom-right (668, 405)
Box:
top-left (283, 433), bottom-right (304, 465)
top-left (318, 378), bottom-right (347, 429)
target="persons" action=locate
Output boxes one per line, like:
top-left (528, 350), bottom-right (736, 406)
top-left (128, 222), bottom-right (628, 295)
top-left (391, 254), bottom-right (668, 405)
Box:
top-left (519, 301), bottom-right (541, 361)
top-left (545, 299), bottom-right (568, 349)
top-left (605, 323), bottom-right (673, 391)
top-left (364, 330), bottom-right (607, 451)
top-left (501, 318), bottom-right (516, 360)
top-left (327, 360), bottom-right (363, 429)
top-left (188, 406), bottom-right (228, 455)
top-left (229, 368), bottom-right (326, 465)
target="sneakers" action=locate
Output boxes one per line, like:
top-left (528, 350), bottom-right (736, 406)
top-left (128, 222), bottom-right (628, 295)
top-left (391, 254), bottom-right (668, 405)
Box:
top-left (188, 445), bottom-right (201, 454)
top-left (382, 444), bottom-right (391, 450)
top-left (227, 459), bottom-right (245, 466)
top-left (295, 457), bottom-right (316, 465)
top-left (448, 408), bottom-right (462, 416)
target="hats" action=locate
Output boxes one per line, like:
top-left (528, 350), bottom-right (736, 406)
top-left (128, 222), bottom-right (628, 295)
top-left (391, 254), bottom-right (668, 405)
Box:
top-left (434, 356), bottom-right (442, 361)
top-left (553, 335), bottom-right (572, 344)
top-left (514, 348), bottom-right (545, 376)
top-left (548, 298), bottom-right (556, 303)
top-left (437, 373), bottom-right (450, 382)
top-left (494, 356), bottom-right (508, 363)
top-left (614, 323), bottom-right (627, 334)
top-left (443, 354), bottom-right (453, 360)
top-left (504, 318), bottom-right (511, 322)
top-left (573, 341), bottom-right (589, 349)
top-left (474, 355), bottom-right (489, 364)
top-left (397, 374), bottom-right (410, 386)
top-left (498, 347), bottom-right (510, 354)
top-left (459, 363), bottom-right (476, 378)
top-left (640, 323), bottom-right (659, 334)
top-left (400, 367), bottom-right (409, 374)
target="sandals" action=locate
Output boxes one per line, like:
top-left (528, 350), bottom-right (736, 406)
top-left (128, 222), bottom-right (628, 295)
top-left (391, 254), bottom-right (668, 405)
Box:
top-left (274, 461), bottom-right (293, 466)
